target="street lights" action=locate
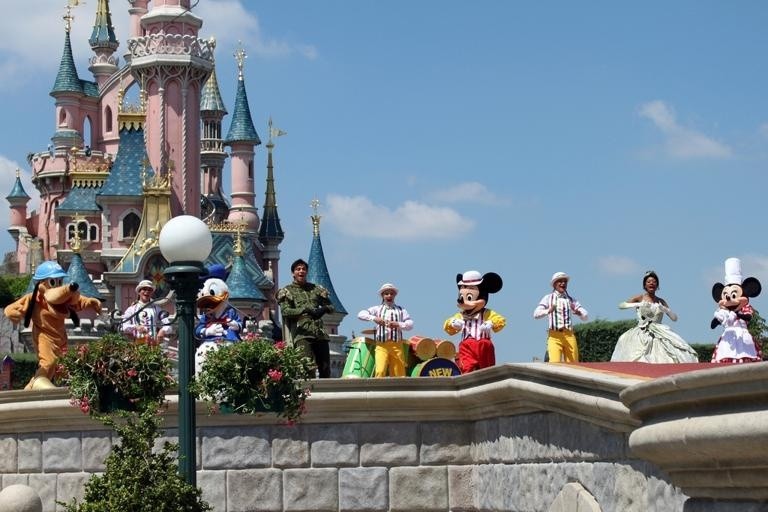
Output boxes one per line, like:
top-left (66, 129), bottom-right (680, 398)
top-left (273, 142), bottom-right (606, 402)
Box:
top-left (158, 214), bottom-right (212, 489)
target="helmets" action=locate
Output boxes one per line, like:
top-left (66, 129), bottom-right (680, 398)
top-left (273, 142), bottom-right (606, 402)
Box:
top-left (33, 261), bottom-right (70, 283)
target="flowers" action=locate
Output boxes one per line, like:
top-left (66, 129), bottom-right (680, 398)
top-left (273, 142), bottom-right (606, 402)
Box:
top-left (194, 332), bottom-right (311, 423)
top-left (53, 330), bottom-right (176, 413)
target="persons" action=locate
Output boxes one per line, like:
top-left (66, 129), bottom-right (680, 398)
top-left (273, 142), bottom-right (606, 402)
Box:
top-left (116, 277), bottom-right (174, 343)
top-left (530, 271), bottom-right (590, 362)
top-left (608, 269), bottom-right (698, 364)
top-left (274, 258), bottom-right (338, 379)
top-left (354, 282), bottom-right (414, 380)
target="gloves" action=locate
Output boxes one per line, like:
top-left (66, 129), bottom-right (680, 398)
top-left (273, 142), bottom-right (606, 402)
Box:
top-left (302, 306), bottom-right (317, 316)
top-left (314, 306), bottom-right (329, 319)
top-left (451, 319), bottom-right (465, 330)
top-left (226, 317), bottom-right (239, 331)
top-left (715, 309), bottom-right (728, 322)
top-left (480, 320), bottom-right (493, 332)
top-left (85, 298), bottom-right (102, 315)
top-left (657, 302), bottom-right (677, 322)
top-left (5, 304), bottom-right (22, 321)
top-left (620, 303), bottom-right (651, 309)
top-left (205, 323), bottom-right (223, 337)
top-left (722, 312), bottom-right (737, 327)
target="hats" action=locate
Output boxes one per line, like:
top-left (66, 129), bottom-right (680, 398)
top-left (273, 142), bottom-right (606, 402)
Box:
top-left (550, 272), bottom-right (569, 289)
top-left (135, 279), bottom-right (156, 295)
top-left (723, 257), bottom-right (741, 286)
top-left (457, 271), bottom-right (483, 286)
top-left (377, 284), bottom-right (399, 297)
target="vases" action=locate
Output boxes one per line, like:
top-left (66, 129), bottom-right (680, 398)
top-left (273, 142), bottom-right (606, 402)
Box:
top-left (220, 395), bottom-right (287, 414)
top-left (98, 392), bottom-right (152, 413)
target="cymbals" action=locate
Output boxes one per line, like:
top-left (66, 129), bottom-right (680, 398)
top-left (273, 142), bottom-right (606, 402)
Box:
top-left (361, 330), bottom-right (376, 333)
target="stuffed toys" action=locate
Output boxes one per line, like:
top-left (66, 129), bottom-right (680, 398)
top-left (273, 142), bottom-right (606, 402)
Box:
top-left (706, 274), bottom-right (765, 365)
top-left (188, 263), bottom-right (243, 380)
top-left (441, 270), bottom-right (509, 371)
top-left (3, 260), bottom-right (104, 393)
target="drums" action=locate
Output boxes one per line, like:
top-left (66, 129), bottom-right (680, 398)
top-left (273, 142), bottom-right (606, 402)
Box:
top-left (409, 336), bottom-right (436, 361)
top-left (432, 338), bottom-right (456, 360)
top-left (410, 357), bottom-right (462, 376)
top-left (342, 337), bottom-right (375, 378)
top-left (402, 339), bottom-right (416, 377)
